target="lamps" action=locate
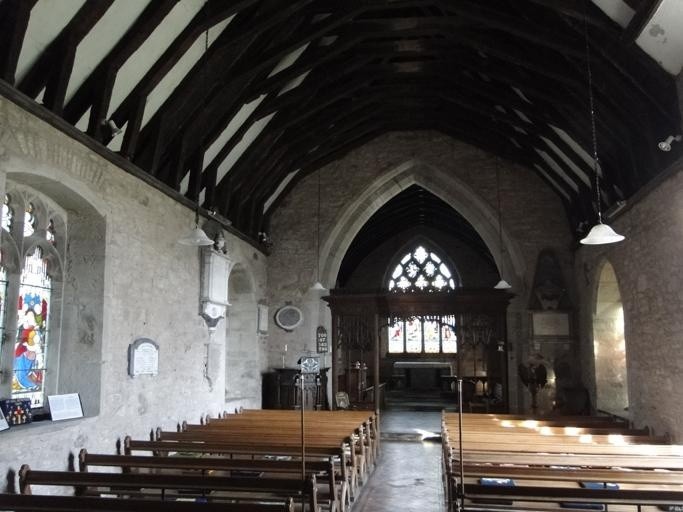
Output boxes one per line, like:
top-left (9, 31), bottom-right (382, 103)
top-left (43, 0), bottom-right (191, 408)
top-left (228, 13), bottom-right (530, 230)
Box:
top-left (576, 8), bottom-right (633, 245)
top-left (100, 117), bottom-right (124, 137)
top-left (493, 153), bottom-right (512, 290)
top-left (657, 133), bottom-right (681, 153)
top-left (258, 231), bottom-right (268, 241)
top-left (176, 27), bottom-right (216, 246)
top-left (308, 159), bottom-right (328, 291)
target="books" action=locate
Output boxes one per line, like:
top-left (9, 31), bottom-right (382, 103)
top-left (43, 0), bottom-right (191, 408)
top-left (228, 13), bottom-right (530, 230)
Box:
top-left (46, 392), bottom-right (85, 422)
top-left (559, 503), bottom-right (603, 510)
top-left (578, 482), bottom-right (618, 491)
top-left (479, 477), bottom-right (514, 489)
top-left (471, 499), bottom-right (514, 506)
top-left (99, 453), bottom-right (337, 506)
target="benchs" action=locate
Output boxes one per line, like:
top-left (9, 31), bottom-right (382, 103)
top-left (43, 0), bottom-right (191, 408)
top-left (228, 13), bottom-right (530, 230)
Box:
top-left (0, 406), bottom-right (382, 511)
top-left (439, 407), bottom-right (683, 512)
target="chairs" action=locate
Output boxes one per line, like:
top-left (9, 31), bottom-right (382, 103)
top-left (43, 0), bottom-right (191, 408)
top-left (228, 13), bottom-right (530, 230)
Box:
top-left (551, 387), bottom-right (590, 415)
